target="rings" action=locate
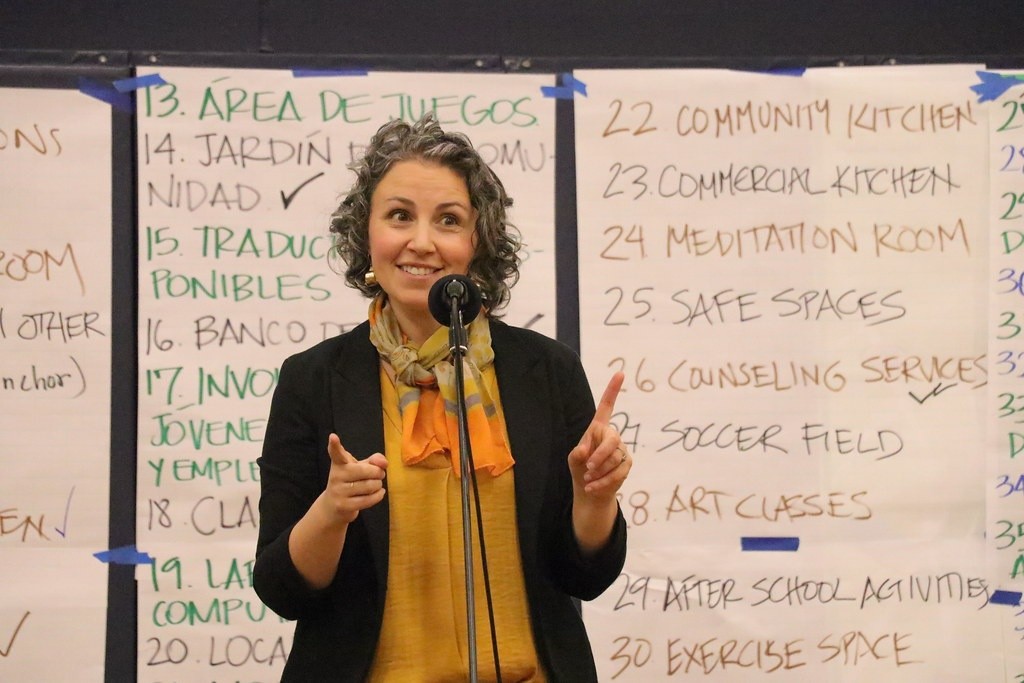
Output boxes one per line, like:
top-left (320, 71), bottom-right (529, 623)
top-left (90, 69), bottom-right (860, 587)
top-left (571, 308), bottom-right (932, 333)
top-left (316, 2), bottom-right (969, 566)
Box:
top-left (616, 447), bottom-right (626, 462)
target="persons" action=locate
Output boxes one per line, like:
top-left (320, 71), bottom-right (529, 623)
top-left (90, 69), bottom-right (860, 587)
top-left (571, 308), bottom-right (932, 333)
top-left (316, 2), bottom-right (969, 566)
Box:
top-left (253, 111), bottom-right (633, 683)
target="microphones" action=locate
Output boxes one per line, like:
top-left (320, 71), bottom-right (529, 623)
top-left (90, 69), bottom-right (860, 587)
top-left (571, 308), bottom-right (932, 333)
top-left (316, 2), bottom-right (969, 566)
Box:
top-left (428, 273), bottom-right (482, 327)
